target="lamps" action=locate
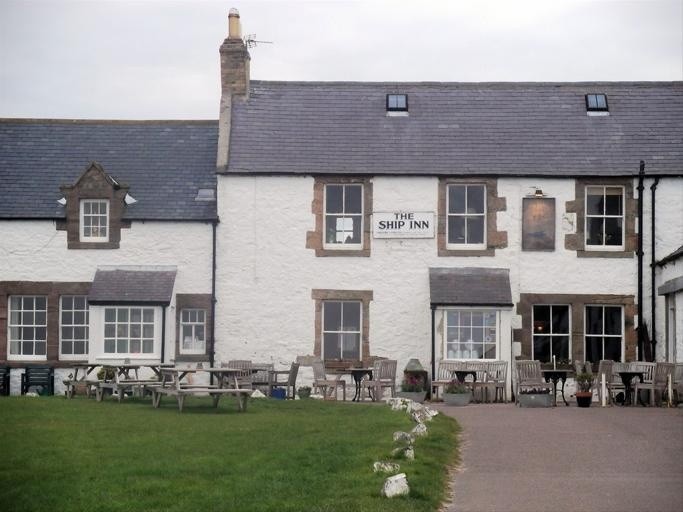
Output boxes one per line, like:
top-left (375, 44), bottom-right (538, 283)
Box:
top-left (533, 190), bottom-right (543, 198)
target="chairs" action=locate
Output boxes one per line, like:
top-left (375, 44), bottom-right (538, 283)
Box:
top-left (428, 353), bottom-right (680, 411)
top-left (4, 354), bottom-right (400, 409)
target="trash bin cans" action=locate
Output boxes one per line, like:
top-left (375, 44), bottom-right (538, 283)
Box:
top-left (273, 388), bottom-right (285, 399)
